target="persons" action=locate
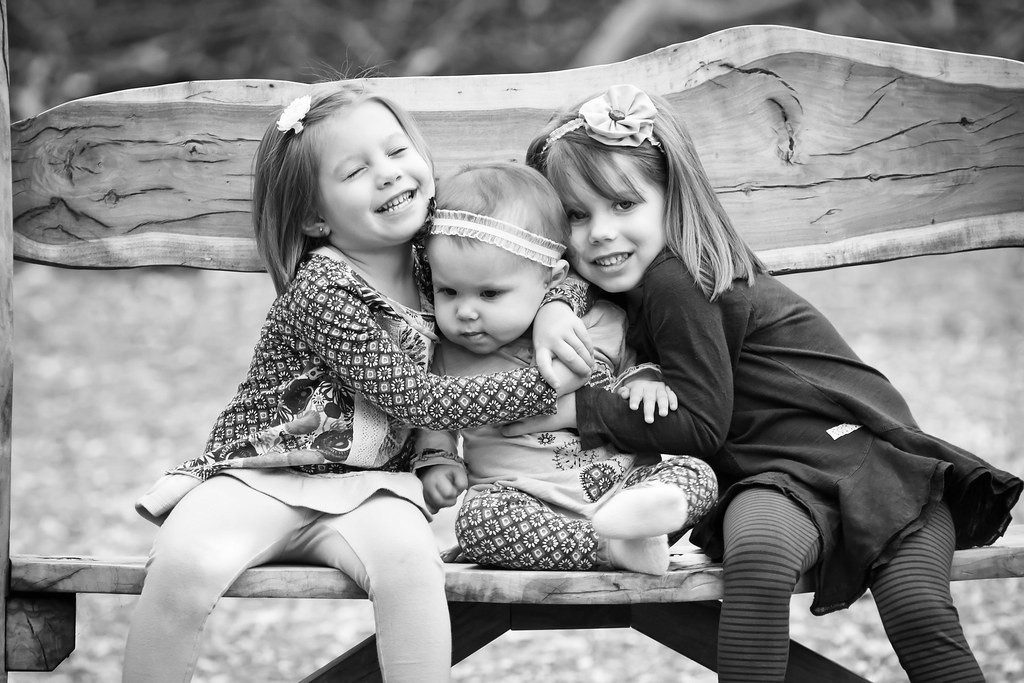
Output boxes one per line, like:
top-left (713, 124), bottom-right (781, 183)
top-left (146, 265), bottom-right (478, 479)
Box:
top-left (121, 79), bottom-right (597, 683)
top-left (407, 162), bottom-right (719, 577)
top-left (491, 87), bottom-right (1024, 683)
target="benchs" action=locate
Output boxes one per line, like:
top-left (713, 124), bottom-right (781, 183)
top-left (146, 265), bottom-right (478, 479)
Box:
top-left (0, 25), bottom-right (1024, 683)
